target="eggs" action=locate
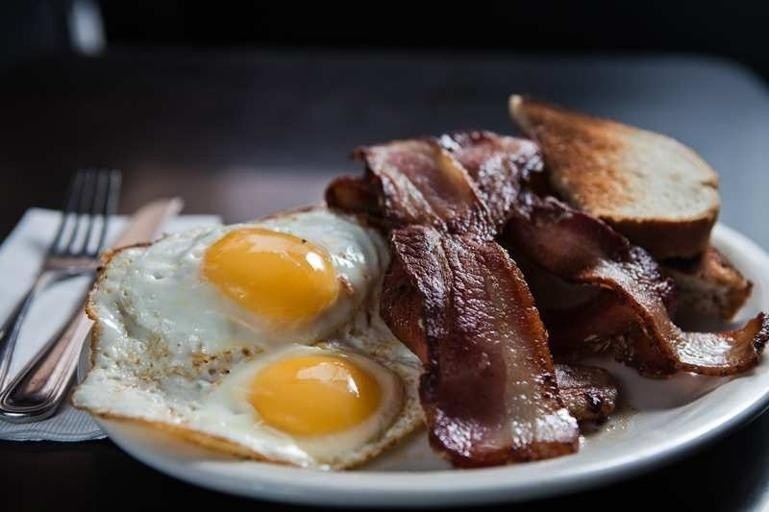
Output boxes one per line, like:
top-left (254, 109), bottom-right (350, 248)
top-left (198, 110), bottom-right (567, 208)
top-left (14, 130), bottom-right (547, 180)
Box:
top-left (84, 206), bottom-right (390, 347)
top-left (68, 334), bottom-right (431, 472)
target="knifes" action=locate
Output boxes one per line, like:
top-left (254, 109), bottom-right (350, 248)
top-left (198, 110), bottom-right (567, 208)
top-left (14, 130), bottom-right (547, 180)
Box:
top-left (2, 194), bottom-right (185, 423)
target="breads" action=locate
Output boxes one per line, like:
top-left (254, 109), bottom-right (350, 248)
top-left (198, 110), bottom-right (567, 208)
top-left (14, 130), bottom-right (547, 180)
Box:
top-left (506, 95), bottom-right (719, 257)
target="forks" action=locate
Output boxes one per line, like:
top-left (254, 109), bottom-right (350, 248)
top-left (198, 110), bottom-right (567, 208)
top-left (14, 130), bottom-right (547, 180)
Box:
top-left (0, 165), bottom-right (123, 395)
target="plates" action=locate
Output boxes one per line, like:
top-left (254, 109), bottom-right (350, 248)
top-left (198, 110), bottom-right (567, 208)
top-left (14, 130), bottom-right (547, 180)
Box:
top-left (85, 214), bottom-right (769, 511)
